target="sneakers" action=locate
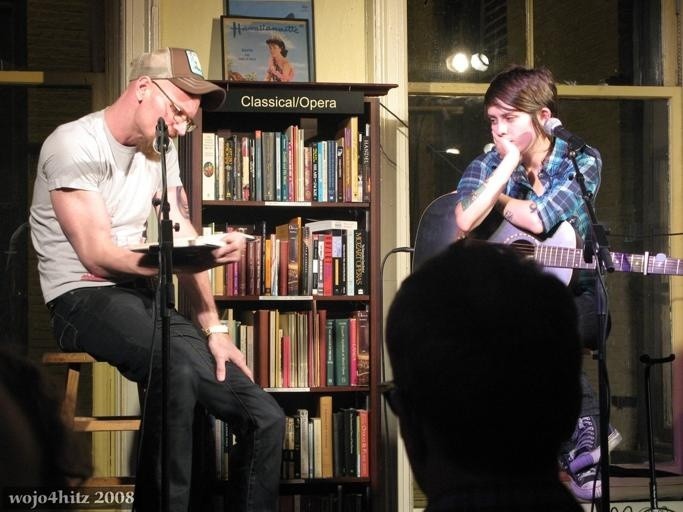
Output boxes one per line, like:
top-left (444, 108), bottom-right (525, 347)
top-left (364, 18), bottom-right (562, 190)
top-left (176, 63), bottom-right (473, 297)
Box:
top-left (558, 416), bottom-right (622, 501)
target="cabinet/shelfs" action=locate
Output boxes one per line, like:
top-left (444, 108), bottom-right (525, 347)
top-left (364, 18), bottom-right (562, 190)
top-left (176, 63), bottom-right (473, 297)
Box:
top-left (176, 79), bottom-right (399, 512)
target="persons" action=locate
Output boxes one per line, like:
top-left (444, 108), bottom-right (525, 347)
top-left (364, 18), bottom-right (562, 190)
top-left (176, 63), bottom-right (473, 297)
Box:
top-left (27, 47), bottom-right (283, 511)
top-left (263, 34), bottom-right (294, 83)
top-left (0, 350), bottom-right (76, 509)
top-left (381, 236), bottom-right (589, 509)
top-left (454, 64), bottom-right (622, 500)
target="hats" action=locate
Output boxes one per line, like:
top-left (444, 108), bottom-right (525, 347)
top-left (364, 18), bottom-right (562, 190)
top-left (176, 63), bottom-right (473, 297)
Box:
top-left (130, 48), bottom-right (227, 111)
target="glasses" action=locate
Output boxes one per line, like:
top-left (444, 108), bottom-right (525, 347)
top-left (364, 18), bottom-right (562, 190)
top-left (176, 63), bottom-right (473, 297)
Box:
top-left (150, 80), bottom-right (196, 133)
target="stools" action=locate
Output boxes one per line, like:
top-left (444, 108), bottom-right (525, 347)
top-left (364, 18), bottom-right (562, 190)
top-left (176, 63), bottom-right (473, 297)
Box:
top-left (39, 349), bottom-right (145, 512)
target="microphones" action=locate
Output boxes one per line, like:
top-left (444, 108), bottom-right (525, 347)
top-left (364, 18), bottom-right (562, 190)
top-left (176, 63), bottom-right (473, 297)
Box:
top-left (151, 125), bottom-right (174, 154)
top-left (543, 117), bottom-right (596, 162)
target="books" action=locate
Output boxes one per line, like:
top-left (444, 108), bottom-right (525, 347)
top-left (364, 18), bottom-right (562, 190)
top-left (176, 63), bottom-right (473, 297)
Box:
top-left (130, 227), bottom-right (255, 255)
top-left (196, 111), bottom-right (374, 511)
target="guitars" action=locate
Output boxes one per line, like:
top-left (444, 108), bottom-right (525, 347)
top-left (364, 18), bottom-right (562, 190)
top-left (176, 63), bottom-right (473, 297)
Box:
top-left (413, 190), bottom-right (683, 287)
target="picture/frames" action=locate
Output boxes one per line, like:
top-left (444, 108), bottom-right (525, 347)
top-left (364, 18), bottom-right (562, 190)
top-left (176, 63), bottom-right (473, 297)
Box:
top-left (219, 0), bottom-right (316, 86)
